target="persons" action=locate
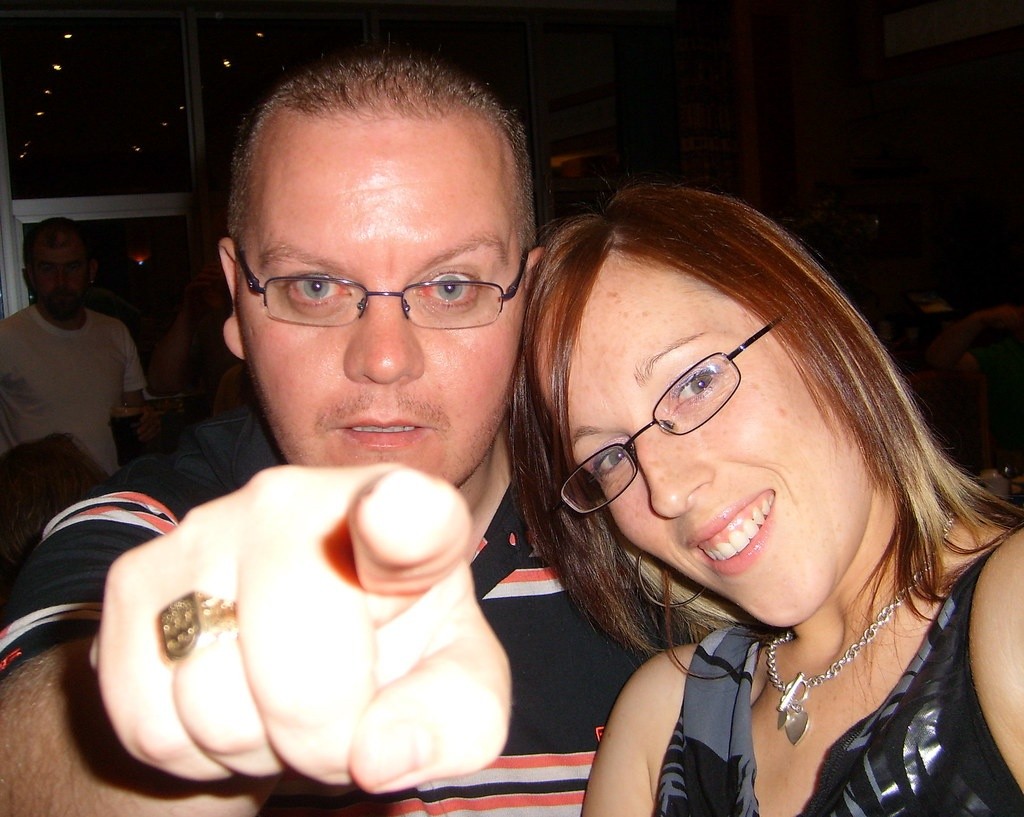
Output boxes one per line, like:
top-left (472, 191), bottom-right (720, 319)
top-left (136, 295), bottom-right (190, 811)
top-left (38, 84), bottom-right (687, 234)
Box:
top-left (1, 43), bottom-right (687, 817)
top-left (493, 173), bottom-right (1020, 815)
top-left (3, 211), bottom-right (163, 579)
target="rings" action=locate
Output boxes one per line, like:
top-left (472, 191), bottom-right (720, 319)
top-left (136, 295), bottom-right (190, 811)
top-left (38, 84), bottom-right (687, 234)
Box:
top-left (152, 591), bottom-right (244, 659)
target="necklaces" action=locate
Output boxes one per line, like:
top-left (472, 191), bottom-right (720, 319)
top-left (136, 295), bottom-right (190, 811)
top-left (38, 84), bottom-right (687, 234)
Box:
top-left (763, 502), bottom-right (959, 748)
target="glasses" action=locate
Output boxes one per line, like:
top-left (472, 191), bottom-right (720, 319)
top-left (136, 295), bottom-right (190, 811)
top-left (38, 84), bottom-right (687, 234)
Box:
top-left (550, 299), bottom-right (781, 523)
top-left (229, 233), bottom-right (530, 336)
